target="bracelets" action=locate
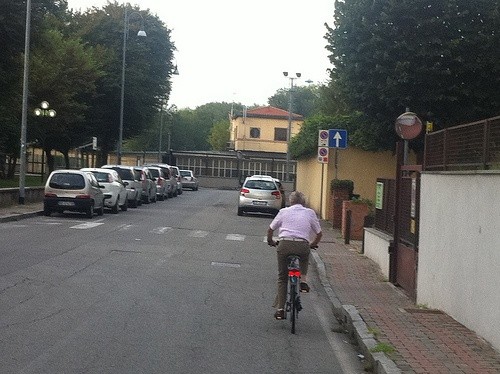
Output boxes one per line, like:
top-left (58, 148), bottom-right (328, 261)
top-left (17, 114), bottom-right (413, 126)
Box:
top-left (268, 240), bottom-right (273, 244)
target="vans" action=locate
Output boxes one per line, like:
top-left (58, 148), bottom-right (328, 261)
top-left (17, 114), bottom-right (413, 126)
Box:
top-left (149, 168), bottom-right (169, 199)
top-left (149, 164), bottom-right (178, 198)
top-left (171, 166), bottom-right (183, 195)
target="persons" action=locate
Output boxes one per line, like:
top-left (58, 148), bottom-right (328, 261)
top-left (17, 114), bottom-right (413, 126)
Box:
top-left (267, 191), bottom-right (322, 320)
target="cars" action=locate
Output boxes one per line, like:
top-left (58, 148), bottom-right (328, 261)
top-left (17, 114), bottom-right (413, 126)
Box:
top-left (103, 163), bottom-right (142, 208)
top-left (179, 170), bottom-right (199, 191)
top-left (82, 167), bottom-right (129, 214)
top-left (274, 179), bottom-right (286, 208)
top-left (43, 170), bottom-right (105, 218)
top-left (238, 177), bottom-right (281, 217)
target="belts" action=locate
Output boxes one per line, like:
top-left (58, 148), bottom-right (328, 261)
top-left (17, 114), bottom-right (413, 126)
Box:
top-left (280, 238), bottom-right (305, 241)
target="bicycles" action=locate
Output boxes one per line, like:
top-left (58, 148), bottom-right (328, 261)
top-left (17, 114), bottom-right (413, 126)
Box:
top-left (264, 241), bottom-right (320, 335)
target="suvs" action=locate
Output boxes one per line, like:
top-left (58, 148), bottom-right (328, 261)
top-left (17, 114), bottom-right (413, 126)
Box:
top-left (134, 167), bottom-right (158, 203)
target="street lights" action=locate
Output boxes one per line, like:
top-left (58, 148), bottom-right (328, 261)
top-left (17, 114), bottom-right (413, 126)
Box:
top-left (158, 61), bottom-right (179, 161)
top-left (33, 101), bottom-right (57, 182)
top-left (305, 79), bottom-right (314, 85)
top-left (282, 71), bottom-right (302, 180)
top-left (118, 11), bottom-right (149, 154)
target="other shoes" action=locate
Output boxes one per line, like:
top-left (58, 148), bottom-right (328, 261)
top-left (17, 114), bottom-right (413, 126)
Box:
top-left (274, 310), bottom-right (286, 320)
top-left (299, 282), bottom-right (310, 292)
